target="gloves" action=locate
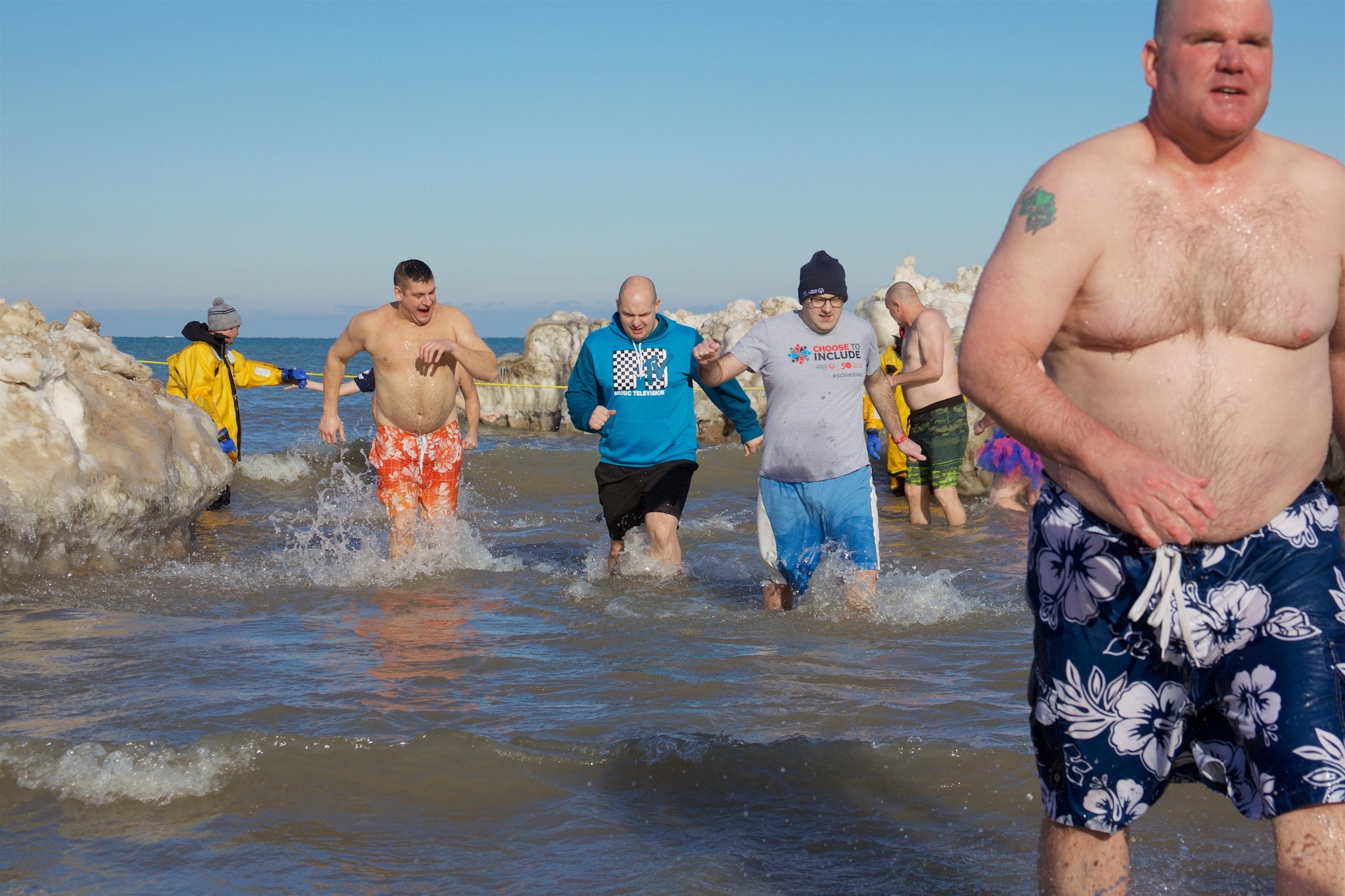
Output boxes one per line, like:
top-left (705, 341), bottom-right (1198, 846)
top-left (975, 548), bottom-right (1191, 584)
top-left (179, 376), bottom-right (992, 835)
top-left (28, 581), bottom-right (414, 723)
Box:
top-left (217, 429), bottom-right (237, 454)
top-left (283, 368), bottom-right (309, 389)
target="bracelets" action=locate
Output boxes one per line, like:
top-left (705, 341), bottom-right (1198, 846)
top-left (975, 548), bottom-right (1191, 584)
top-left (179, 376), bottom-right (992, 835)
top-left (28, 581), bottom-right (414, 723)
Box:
top-left (891, 432), bottom-right (907, 443)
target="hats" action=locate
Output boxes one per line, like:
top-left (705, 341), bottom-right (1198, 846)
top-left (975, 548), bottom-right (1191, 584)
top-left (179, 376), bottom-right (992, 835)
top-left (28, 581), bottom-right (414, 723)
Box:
top-left (798, 251), bottom-right (848, 304)
top-left (208, 298), bottom-right (242, 332)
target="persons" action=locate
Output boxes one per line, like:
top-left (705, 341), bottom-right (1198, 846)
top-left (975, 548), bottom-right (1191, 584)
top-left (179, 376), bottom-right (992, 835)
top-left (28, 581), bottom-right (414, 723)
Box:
top-left (885, 282), bottom-right (970, 525)
top-left (164, 296), bottom-right (310, 511)
top-left (973, 412), bottom-right (1044, 513)
top-left (863, 347), bottom-right (911, 497)
top-left (283, 367), bottom-right (499, 423)
top-left (692, 250), bottom-right (927, 613)
top-left (959, 0), bottom-right (1345, 896)
top-left (317, 261), bottom-right (498, 559)
top-left (564, 275), bottom-right (765, 577)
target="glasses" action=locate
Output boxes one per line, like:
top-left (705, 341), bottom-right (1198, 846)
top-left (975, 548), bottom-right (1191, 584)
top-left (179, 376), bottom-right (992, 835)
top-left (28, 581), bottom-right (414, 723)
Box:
top-left (809, 295), bottom-right (844, 308)
